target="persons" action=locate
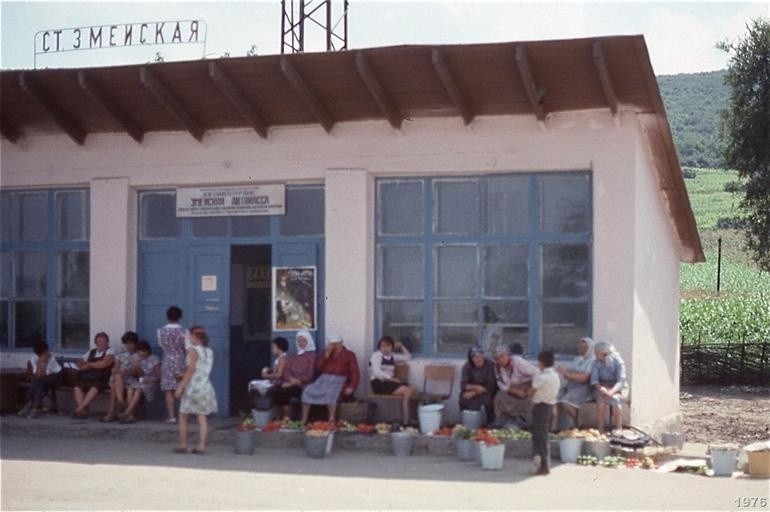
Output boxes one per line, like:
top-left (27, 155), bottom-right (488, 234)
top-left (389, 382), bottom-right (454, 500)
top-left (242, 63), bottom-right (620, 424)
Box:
top-left (72, 332), bottom-right (115, 417)
top-left (156, 305), bottom-right (187, 422)
top-left (460, 306), bottom-right (630, 435)
top-left (274, 332), bottom-right (317, 422)
top-left (300, 333), bottom-right (360, 424)
top-left (17, 341), bottom-right (62, 417)
top-left (100, 331), bottom-right (140, 422)
top-left (528, 349), bottom-right (562, 475)
top-left (247, 337), bottom-right (289, 418)
top-left (369, 336), bottom-right (417, 427)
top-left (116, 339), bottom-right (162, 422)
top-left (275, 275), bottom-right (313, 330)
top-left (174, 327), bottom-right (219, 453)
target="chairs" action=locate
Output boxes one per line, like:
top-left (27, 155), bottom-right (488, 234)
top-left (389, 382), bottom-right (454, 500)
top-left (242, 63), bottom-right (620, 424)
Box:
top-left (410, 364), bottom-right (456, 424)
top-left (369, 363), bottom-right (411, 423)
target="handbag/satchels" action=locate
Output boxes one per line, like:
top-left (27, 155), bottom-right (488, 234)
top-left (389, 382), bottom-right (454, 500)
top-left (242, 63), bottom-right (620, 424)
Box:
top-left (62, 367), bottom-right (80, 387)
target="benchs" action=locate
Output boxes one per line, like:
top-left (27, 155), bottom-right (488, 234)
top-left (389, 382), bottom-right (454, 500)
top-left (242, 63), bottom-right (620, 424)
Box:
top-left (18, 356), bottom-right (147, 419)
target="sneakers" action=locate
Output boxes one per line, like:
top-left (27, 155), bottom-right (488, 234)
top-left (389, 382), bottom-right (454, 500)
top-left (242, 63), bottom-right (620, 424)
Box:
top-left (17, 404), bottom-right (177, 423)
top-left (527, 455), bottom-right (542, 475)
top-left (539, 469), bottom-right (549, 474)
top-left (191, 449), bottom-right (205, 454)
top-left (173, 447), bottom-right (188, 452)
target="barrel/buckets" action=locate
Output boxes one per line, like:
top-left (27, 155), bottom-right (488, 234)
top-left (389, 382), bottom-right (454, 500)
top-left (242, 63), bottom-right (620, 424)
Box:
top-left (234, 432), bottom-right (254, 455)
top-left (585, 440), bottom-right (610, 460)
top-left (711, 449), bottom-right (736, 475)
top-left (662, 432), bottom-right (685, 451)
top-left (392, 432), bottom-right (412, 456)
top-left (305, 429), bottom-right (334, 457)
top-left (418, 404), bottom-right (445, 433)
top-left (560, 438), bottom-right (581, 463)
top-left (480, 440), bottom-right (505, 469)
top-left (462, 410), bottom-right (481, 431)
top-left (455, 439), bottom-right (474, 461)
top-left (748, 451), bottom-right (770, 477)
